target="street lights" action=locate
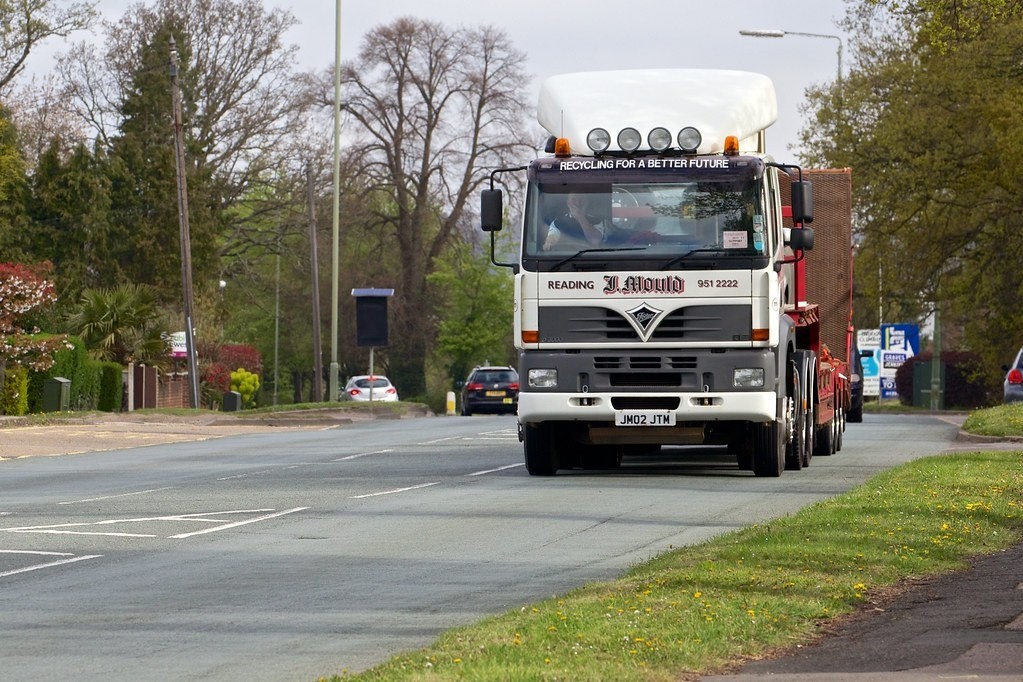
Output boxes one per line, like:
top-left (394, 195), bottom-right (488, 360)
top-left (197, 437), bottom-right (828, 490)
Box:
top-left (737, 27), bottom-right (845, 167)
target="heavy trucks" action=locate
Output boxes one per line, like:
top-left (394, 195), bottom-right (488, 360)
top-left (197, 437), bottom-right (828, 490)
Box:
top-left (477, 67), bottom-right (857, 479)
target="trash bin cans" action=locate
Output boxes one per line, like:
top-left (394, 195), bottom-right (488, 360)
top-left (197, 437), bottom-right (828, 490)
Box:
top-left (913, 361), bottom-right (946, 408)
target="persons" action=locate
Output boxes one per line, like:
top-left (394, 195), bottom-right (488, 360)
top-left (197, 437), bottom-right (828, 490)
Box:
top-left (543, 188), bottom-right (607, 251)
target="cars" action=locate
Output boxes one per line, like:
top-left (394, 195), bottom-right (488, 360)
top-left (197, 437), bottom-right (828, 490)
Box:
top-left (843, 342), bottom-right (875, 424)
top-left (1000, 345), bottom-right (1022, 405)
top-left (455, 364), bottom-right (520, 417)
top-left (337, 374), bottom-right (399, 403)
